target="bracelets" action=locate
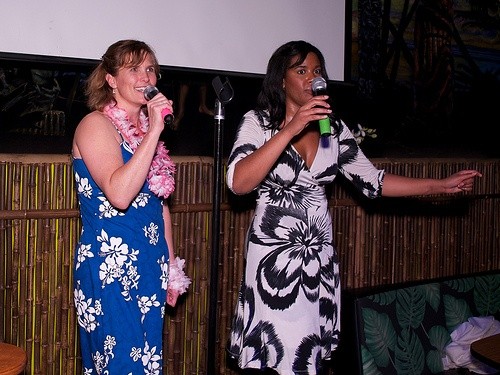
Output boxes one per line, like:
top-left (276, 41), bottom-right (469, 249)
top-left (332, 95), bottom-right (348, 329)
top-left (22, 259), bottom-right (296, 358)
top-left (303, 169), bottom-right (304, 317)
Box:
top-left (167, 256), bottom-right (191, 295)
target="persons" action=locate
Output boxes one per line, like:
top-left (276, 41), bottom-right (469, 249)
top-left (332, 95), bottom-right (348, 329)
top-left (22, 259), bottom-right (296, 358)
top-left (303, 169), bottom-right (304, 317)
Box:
top-left (225, 40), bottom-right (484, 375)
top-left (68, 34), bottom-right (191, 375)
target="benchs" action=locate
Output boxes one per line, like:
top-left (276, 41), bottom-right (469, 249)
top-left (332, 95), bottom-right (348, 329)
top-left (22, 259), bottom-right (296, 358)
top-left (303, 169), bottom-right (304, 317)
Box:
top-left (340, 270), bottom-right (500, 374)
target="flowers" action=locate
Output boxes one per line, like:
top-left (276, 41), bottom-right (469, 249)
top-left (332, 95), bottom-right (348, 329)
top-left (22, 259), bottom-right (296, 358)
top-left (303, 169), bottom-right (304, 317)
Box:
top-left (166, 257), bottom-right (190, 296)
top-left (100, 99), bottom-right (176, 199)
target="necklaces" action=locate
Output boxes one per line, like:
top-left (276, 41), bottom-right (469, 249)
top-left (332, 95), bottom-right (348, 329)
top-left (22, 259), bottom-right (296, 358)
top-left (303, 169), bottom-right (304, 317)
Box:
top-left (99, 100), bottom-right (178, 200)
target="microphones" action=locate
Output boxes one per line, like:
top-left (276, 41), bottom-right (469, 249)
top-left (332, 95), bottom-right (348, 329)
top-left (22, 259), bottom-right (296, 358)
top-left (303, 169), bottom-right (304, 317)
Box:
top-left (144, 86), bottom-right (174, 124)
top-left (312, 76), bottom-right (332, 136)
